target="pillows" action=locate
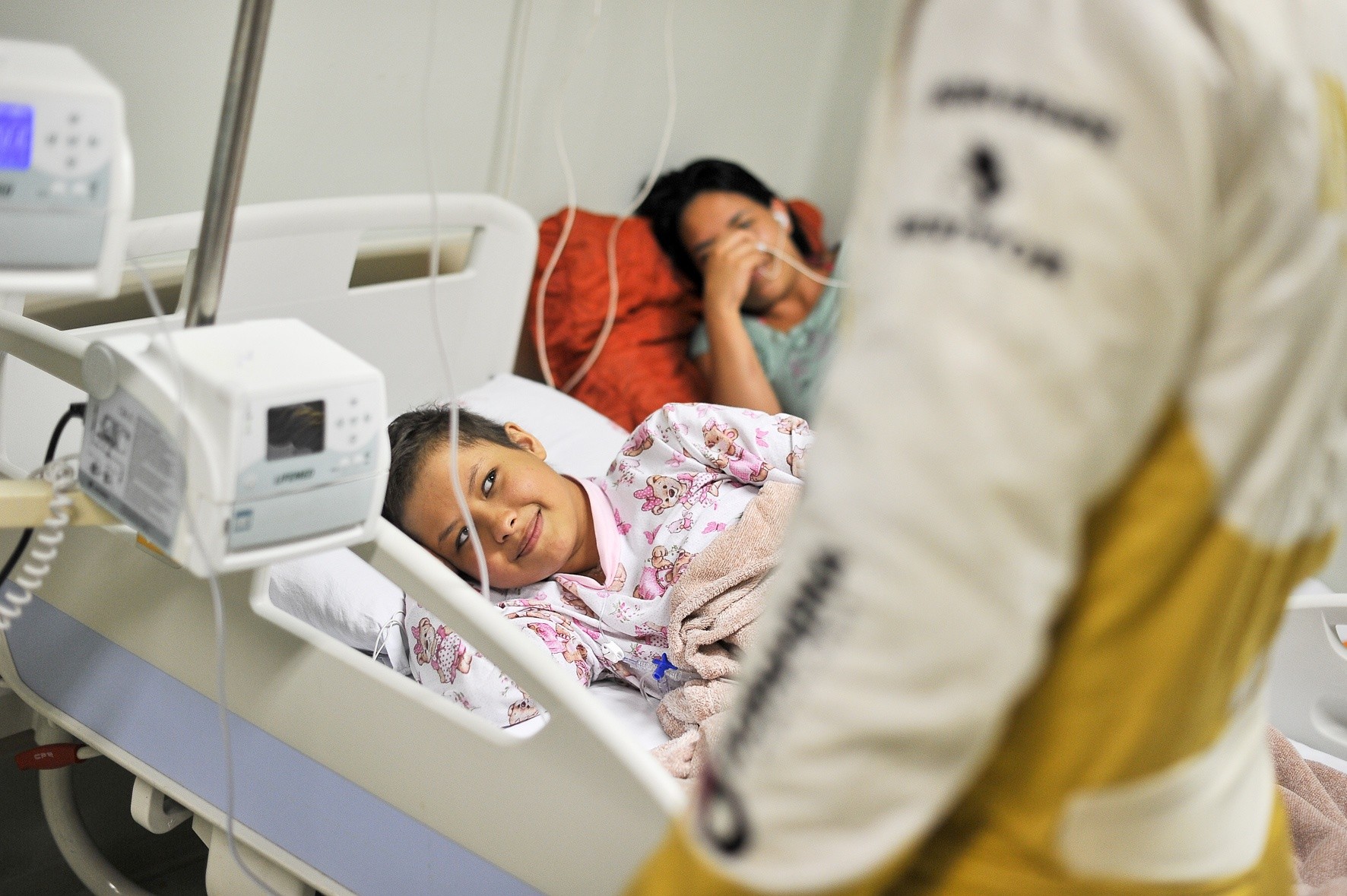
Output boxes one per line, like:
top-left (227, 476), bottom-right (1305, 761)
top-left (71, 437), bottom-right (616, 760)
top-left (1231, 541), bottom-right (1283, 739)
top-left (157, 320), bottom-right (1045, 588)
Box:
top-left (266, 376), bottom-right (631, 656)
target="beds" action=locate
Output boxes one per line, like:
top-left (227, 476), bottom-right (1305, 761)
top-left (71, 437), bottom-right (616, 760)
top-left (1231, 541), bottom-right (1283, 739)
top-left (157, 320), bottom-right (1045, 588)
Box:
top-left (0, 193), bottom-right (1345, 896)
top-left (531, 201), bottom-right (838, 431)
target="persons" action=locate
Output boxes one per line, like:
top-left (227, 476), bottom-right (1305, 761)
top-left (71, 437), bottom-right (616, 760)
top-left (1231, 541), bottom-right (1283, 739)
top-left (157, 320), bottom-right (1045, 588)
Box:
top-left (649, 159), bottom-right (846, 427)
top-left (377, 400), bottom-right (814, 731)
top-left (619, 0), bottom-right (1347, 894)
